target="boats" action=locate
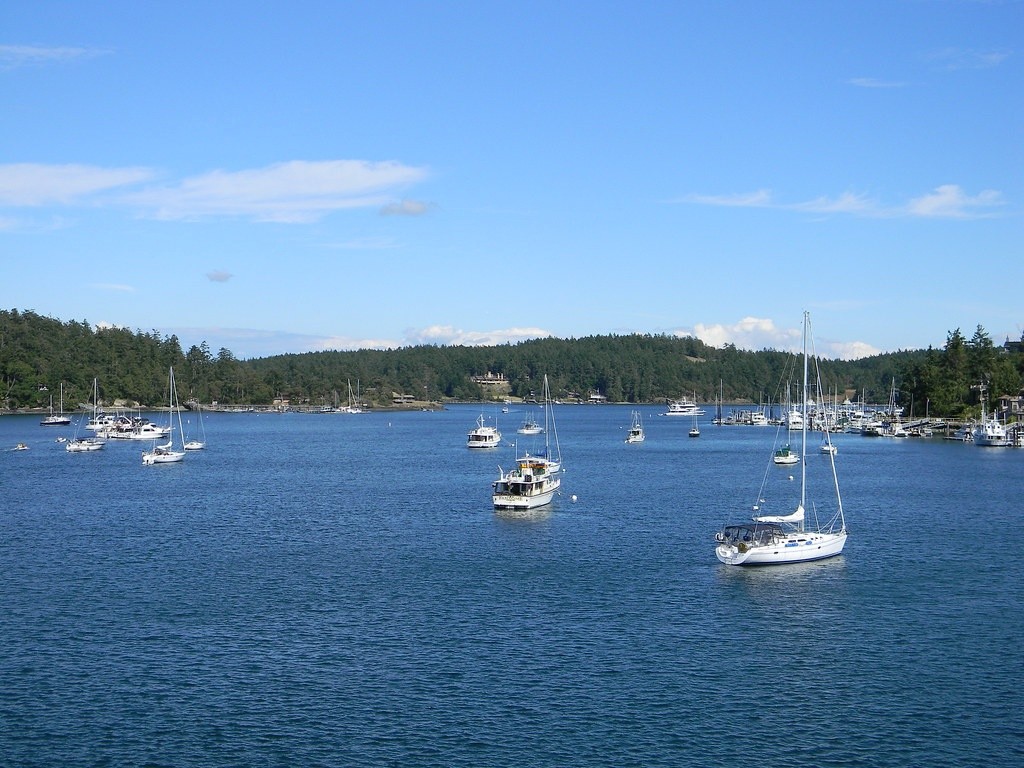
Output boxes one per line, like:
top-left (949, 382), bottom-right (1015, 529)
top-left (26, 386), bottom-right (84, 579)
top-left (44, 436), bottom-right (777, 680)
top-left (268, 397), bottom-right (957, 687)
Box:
top-left (466, 413), bottom-right (502, 449)
top-left (625, 409), bottom-right (647, 444)
top-left (501, 407), bottom-right (508, 414)
top-left (663, 396), bottom-right (706, 417)
top-left (517, 411), bottom-right (544, 436)
top-left (490, 461), bottom-right (562, 512)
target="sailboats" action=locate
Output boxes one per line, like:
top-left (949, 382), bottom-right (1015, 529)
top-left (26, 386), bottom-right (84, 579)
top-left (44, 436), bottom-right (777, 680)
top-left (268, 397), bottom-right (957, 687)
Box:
top-left (773, 384), bottom-right (800, 464)
top-left (345, 378), bottom-right (363, 414)
top-left (689, 390), bottom-right (700, 438)
top-left (67, 365), bottom-right (206, 464)
top-left (708, 372), bottom-right (1024, 456)
top-left (514, 374), bottom-right (562, 473)
top-left (40, 382), bottom-right (71, 426)
top-left (716, 310), bottom-right (849, 566)
